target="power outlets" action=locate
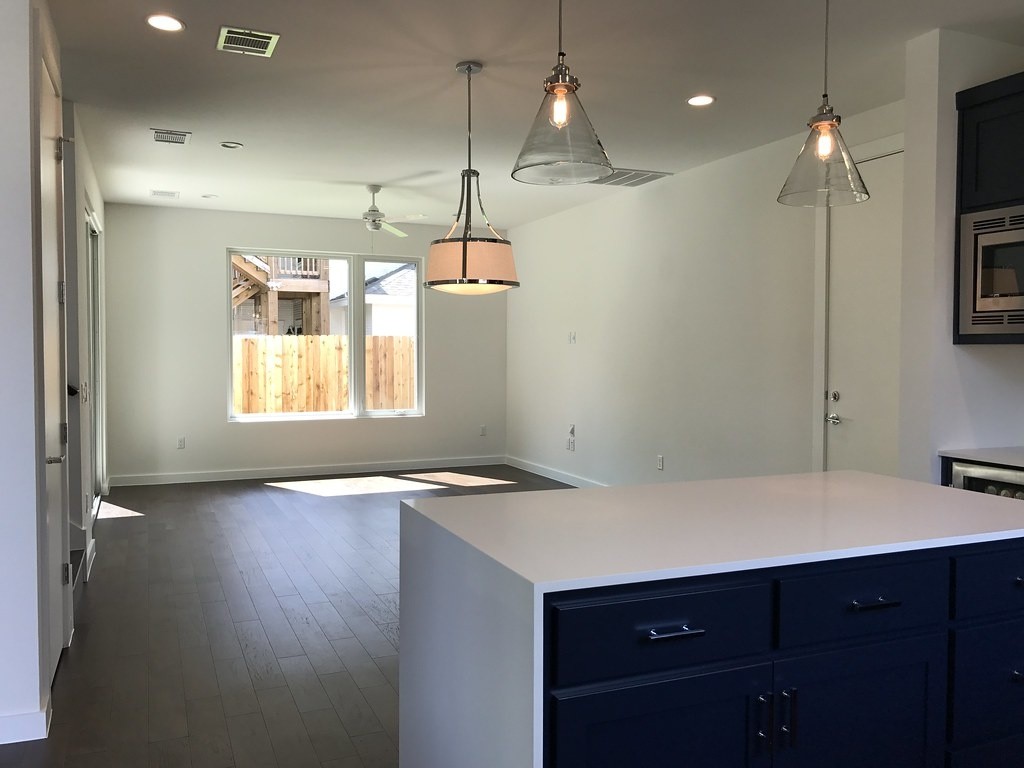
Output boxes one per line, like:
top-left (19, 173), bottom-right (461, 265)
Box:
top-left (657, 455), bottom-right (663, 471)
top-left (177, 436), bottom-right (185, 449)
top-left (565, 437), bottom-right (570, 450)
top-left (570, 438), bottom-right (575, 451)
top-left (480, 425), bottom-right (487, 436)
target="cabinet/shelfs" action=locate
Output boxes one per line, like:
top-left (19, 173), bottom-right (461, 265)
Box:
top-left (399, 470), bottom-right (1024, 768)
top-left (952, 70), bottom-right (1024, 343)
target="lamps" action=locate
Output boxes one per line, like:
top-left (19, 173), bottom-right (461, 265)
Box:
top-left (422, 61), bottom-right (521, 296)
top-left (511, 0), bottom-right (615, 187)
top-left (777, 0), bottom-right (871, 208)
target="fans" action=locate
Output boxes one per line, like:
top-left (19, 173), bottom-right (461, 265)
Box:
top-left (362, 184), bottom-right (430, 239)
